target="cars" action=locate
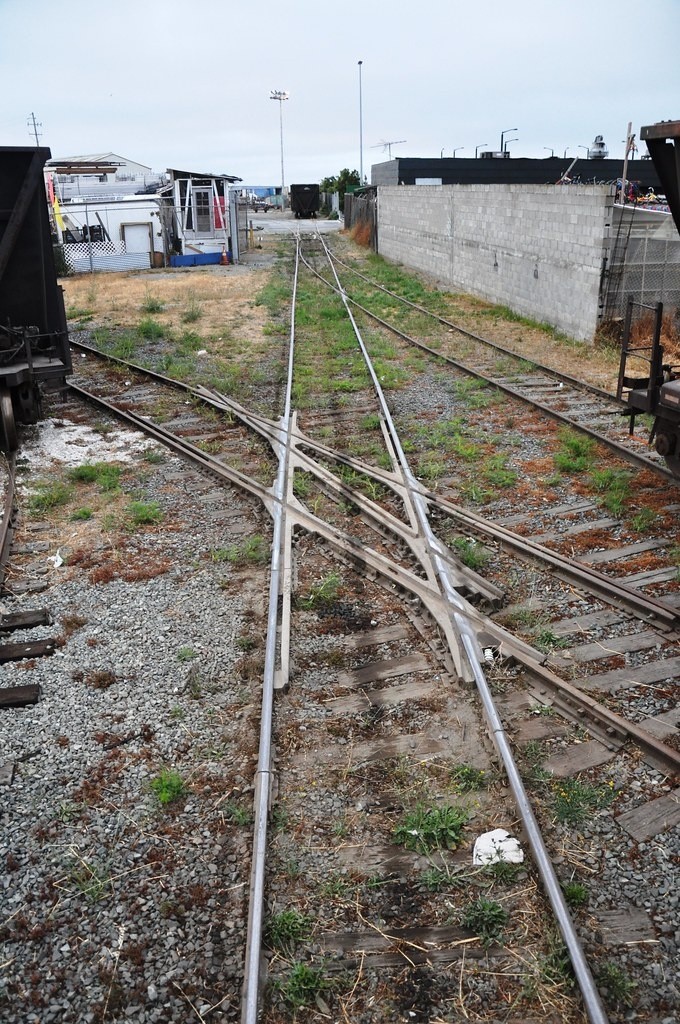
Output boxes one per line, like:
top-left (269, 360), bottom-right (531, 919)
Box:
top-left (265, 194), bottom-right (289, 210)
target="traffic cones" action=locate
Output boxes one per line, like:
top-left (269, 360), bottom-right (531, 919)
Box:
top-left (220, 244), bottom-right (228, 265)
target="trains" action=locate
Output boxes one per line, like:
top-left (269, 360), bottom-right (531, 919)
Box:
top-left (290, 183), bottom-right (320, 219)
top-left (1, 146), bottom-right (77, 451)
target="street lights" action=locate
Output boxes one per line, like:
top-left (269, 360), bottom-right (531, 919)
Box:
top-left (501, 128), bottom-right (518, 152)
top-left (504, 139), bottom-right (518, 152)
top-left (453, 147), bottom-right (464, 158)
top-left (358, 60), bottom-right (363, 187)
top-left (269, 89), bottom-right (289, 213)
top-left (475, 143), bottom-right (487, 159)
